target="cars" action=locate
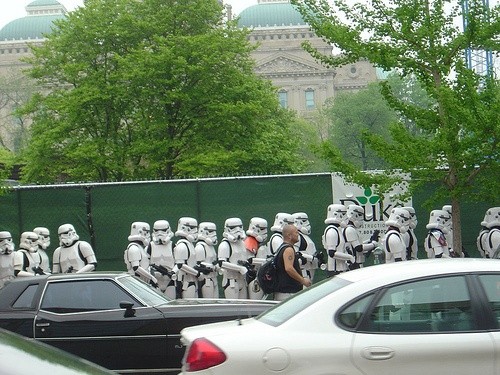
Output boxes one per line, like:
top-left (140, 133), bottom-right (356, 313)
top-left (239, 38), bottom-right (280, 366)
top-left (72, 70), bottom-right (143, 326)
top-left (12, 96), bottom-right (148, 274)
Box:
top-left (0, 272), bottom-right (282, 375)
top-left (180, 258), bottom-right (500, 375)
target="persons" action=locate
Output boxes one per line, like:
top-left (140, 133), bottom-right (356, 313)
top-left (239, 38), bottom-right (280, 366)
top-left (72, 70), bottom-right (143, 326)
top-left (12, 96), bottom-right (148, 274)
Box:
top-left (33, 227), bottom-right (51, 274)
top-left (290, 211), bottom-right (327, 290)
top-left (440, 205), bottom-right (467, 257)
top-left (0, 231), bottom-right (18, 285)
top-left (343, 206), bottom-right (379, 270)
top-left (143, 220), bottom-right (178, 300)
top-left (477, 208), bottom-right (491, 258)
top-left (424, 209), bottom-right (460, 258)
top-left (402, 207), bottom-right (418, 260)
top-left (52, 223), bottom-right (98, 275)
top-left (271, 224), bottom-right (312, 301)
top-left (174, 217), bottom-right (209, 298)
top-left (486, 207), bottom-right (500, 259)
top-left (381, 209), bottom-right (411, 265)
top-left (124, 222), bottom-right (158, 290)
top-left (13, 231), bottom-right (51, 277)
top-left (245, 217), bottom-right (273, 301)
top-left (195, 222), bottom-right (223, 299)
top-left (321, 204), bottom-right (356, 275)
top-left (267, 212), bottom-right (305, 302)
top-left (217, 217), bottom-right (250, 299)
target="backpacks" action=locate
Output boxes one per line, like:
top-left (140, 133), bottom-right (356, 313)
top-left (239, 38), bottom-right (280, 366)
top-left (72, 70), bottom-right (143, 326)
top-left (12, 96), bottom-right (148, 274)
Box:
top-left (258, 243), bottom-right (294, 294)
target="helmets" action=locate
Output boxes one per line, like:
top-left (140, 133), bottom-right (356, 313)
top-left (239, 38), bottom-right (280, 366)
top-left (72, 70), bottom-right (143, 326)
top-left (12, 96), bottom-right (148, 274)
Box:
top-left (152, 220), bottom-right (174, 245)
top-left (198, 222), bottom-right (218, 246)
top-left (127, 222), bottom-right (151, 247)
top-left (19, 232), bottom-right (39, 252)
top-left (175, 217), bottom-right (199, 243)
top-left (33, 227), bottom-right (51, 249)
top-left (58, 223), bottom-right (80, 247)
top-left (385, 205), bottom-right (500, 234)
top-left (0, 231), bottom-right (15, 254)
top-left (347, 205), bottom-right (365, 229)
top-left (247, 217), bottom-right (268, 242)
top-left (223, 218), bottom-right (246, 242)
top-left (270, 213), bottom-right (295, 233)
top-left (293, 212), bottom-right (311, 234)
top-left (324, 204), bottom-right (348, 229)
